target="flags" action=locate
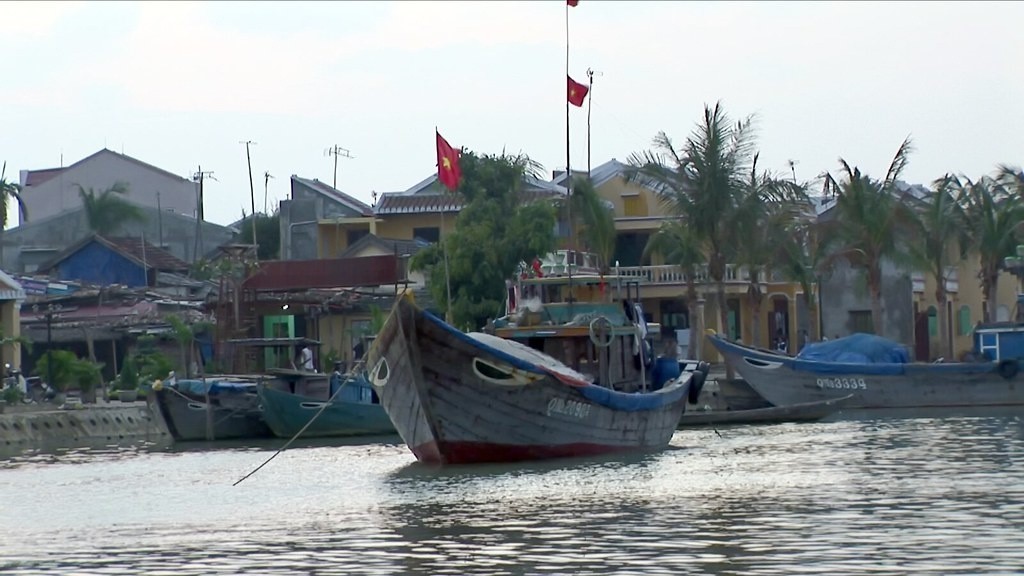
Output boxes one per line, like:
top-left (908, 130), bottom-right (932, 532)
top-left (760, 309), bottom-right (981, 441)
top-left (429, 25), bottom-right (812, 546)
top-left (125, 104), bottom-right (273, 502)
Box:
top-left (567, 75), bottom-right (588, 107)
top-left (436, 133), bottom-right (461, 192)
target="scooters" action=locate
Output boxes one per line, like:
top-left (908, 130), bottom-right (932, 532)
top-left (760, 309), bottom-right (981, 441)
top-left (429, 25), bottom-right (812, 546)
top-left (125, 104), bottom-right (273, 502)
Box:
top-left (1, 363), bottom-right (56, 404)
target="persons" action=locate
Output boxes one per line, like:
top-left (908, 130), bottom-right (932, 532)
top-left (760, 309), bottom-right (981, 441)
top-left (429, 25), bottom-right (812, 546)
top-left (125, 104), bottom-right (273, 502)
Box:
top-left (301, 348), bottom-right (313, 371)
top-left (518, 257), bottom-right (543, 278)
top-left (777, 331), bottom-right (840, 352)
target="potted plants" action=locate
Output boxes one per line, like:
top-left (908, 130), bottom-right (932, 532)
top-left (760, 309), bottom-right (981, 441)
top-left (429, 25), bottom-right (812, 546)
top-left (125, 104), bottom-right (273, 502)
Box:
top-left (35, 349), bottom-right (79, 406)
top-left (117, 352), bottom-right (139, 403)
top-left (75, 357), bottom-right (106, 404)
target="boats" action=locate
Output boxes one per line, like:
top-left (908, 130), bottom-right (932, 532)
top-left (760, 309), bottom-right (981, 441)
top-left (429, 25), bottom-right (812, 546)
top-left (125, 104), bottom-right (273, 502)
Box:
top-left (680, 392), bottom-right (855, 425)
top-left (363, 246), bottom-right (712, 469)
top-left (149, 378), bottom-right (275, 442)
top-left (704, 327), bottom-right (1024, 410)
top-left (256, 365), bottom-right (397, 438)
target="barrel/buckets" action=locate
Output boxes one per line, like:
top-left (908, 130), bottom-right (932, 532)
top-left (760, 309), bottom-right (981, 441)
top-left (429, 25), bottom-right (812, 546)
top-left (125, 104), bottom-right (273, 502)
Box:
top-left (652, 358), bottom-right (679, 390)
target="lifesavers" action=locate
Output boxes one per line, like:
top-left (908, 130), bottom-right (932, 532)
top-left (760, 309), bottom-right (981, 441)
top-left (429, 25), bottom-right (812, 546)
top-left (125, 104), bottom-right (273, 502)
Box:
top-left (997, 357), bottom-right (1019, 378)
top-left (689, 360), bottom-right (713, 406)
